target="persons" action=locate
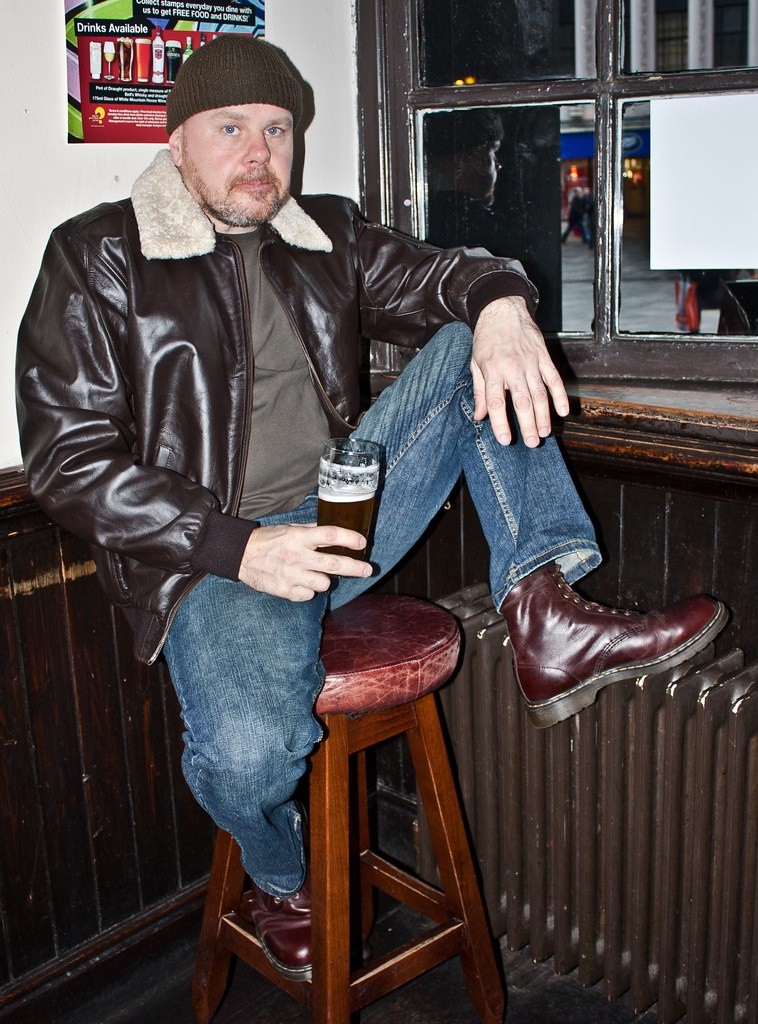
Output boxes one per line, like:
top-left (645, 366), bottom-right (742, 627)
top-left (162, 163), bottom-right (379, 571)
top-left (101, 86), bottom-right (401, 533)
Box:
top-left (560, 188), bottom-right (595, 243)
top-left (418, 107), bottom-right (503, 248)
top-left (15, 33), bottom-right (731, 982)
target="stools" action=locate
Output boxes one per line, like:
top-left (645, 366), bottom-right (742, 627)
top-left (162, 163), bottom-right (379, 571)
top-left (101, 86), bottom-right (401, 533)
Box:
top-left (191, 593), bottom-right (506, 1024)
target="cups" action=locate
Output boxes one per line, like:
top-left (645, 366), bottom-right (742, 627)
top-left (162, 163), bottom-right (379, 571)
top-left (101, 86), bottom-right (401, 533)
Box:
top-left (166, 40), bottom-right (181, 84)
top-left (117, 38), bottom-right (133, 81)
top-left (315, 438), bottom-right (380, 576)
top-left (135, 39), bottom-right (151, 82)
top-left (89, 42), bottom-right (101, 79)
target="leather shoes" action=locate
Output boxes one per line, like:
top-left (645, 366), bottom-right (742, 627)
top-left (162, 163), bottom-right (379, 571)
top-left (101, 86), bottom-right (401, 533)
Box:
top-left (250, 868), bottom-right (312, 981)
top-left (501, 562), bottom-right (730, 729)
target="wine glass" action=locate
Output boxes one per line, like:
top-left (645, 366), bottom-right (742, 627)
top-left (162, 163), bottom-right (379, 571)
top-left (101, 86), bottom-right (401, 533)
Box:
top-left (103, 42), bottom-right (115, 80)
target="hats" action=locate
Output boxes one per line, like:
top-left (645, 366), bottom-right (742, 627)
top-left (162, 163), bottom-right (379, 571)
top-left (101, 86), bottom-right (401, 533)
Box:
top-left (425, 108), bottom-right (505, 153)
top-left (166, 35), bottom-right (304, 136)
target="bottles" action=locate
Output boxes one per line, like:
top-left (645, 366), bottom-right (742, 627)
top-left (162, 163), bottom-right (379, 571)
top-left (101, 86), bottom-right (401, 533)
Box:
top-left (200, 32), bottom-right (205, 48)
top-left (152, 26), bottom-right (164, 84)
top-left (183, 37), bottom-right (193, 64)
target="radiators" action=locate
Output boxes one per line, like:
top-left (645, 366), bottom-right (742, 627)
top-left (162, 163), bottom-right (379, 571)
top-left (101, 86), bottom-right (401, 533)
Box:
top-left (415, 578), bottom-right (758, 1022)
